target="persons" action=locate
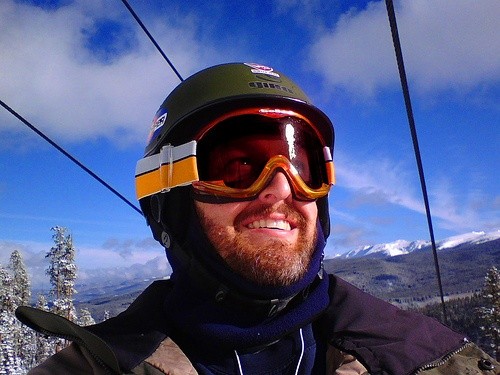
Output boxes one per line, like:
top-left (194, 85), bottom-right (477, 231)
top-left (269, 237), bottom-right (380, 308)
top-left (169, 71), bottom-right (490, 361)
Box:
top-left (25, 60), bottom-right (500, 375)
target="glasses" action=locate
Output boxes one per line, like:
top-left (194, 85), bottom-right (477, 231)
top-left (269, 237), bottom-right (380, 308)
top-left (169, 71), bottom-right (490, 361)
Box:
top-left (186, 106), bottom-right (335, 203)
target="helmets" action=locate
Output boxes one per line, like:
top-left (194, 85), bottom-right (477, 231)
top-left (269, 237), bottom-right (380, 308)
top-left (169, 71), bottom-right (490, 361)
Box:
top-left (138, 61), bottom-right (336, 249)
